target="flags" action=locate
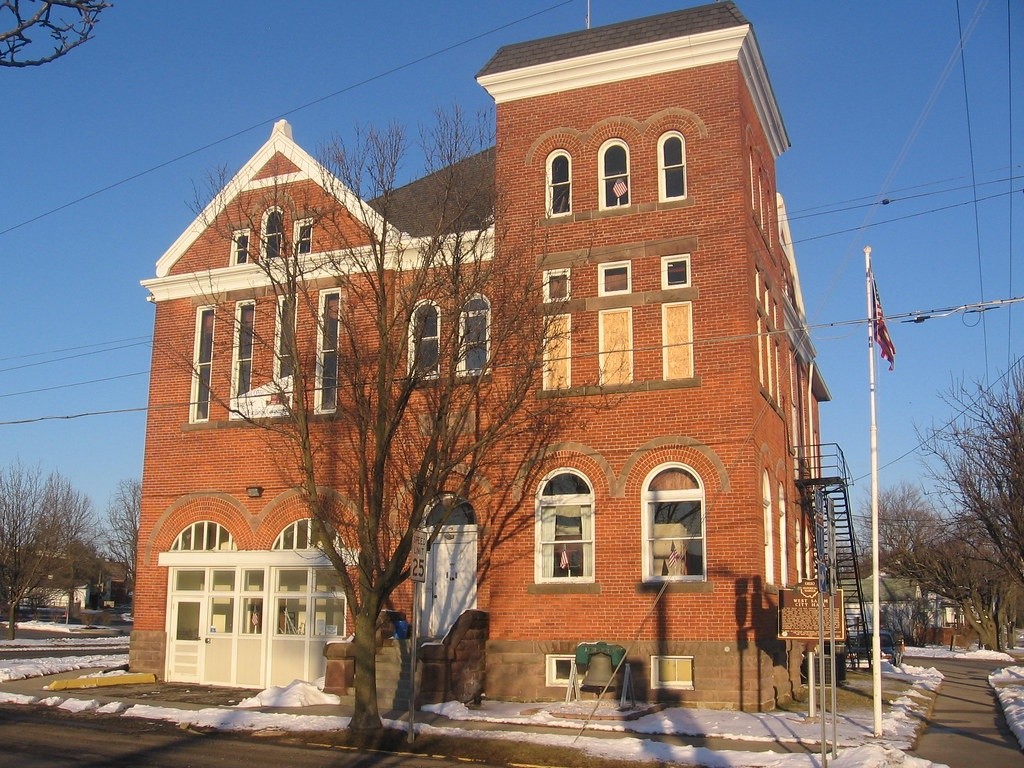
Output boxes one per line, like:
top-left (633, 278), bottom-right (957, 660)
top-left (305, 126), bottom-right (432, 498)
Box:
top-left (560, 544), bottom-right (568, 568)
top-left (870, 259), bottom-right (896, 370)
top-left (668, 542), bottom-right (677, 568)
top-left (252, 604), bottom-right (258, 625)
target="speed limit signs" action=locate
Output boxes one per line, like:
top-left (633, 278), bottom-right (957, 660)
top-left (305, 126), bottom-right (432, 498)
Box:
top-left (409, 529), bottom-right (427, 584)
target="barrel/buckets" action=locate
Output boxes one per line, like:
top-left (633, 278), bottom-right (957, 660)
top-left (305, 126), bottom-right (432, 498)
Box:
top-left (394, 621), bottom-right (408, 640)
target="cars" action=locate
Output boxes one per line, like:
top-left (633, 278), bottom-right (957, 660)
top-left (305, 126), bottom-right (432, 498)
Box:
top-left (845, 629), bottom-right (904, 667)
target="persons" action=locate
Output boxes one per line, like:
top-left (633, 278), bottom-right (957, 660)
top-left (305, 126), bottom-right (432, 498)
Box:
top-left (894, 634), bottom-right (905, 667)
top-left (278, 606), bottom-right (288, 634)
